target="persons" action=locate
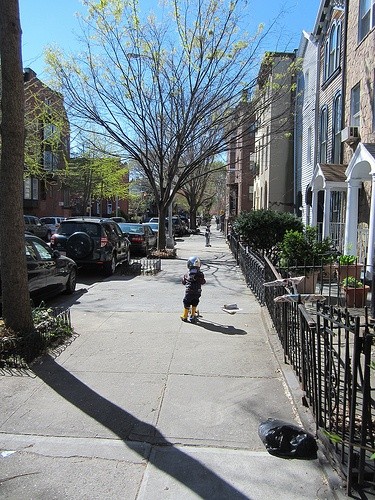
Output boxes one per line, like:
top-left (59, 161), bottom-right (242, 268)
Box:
top-left (180, 257), bottom-right (206, 324)
top-left (205, 224), bottom-right (210, 246)
top-left (196, 220), bottom-right (198, 228)
top-left (215, 217), bottom-right (219, 230)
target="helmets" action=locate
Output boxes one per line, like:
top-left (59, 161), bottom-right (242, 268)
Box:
top-left (187, 257), bottom-right (200, 269)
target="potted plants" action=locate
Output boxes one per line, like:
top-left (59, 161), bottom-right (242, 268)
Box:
top-left (275, 224), bottom-right (370, 308)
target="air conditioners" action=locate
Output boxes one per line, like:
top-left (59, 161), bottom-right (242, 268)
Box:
top-left (341, 126), bottom-right (359, 142)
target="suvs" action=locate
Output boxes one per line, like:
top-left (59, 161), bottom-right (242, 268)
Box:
top-left (49, 216), bottom-right (132, 275)
top-left (23, 215), bottom-right (52, 242)
top-left (40, 216), bottom-right (65, 235)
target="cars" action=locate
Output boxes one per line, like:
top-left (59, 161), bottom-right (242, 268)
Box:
top-left (109, 213), bottom-right (202, 257)
top-left (0, 230), bottom-right (78, 309)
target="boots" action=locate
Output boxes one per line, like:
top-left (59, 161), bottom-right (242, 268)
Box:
top-left (190, 306), bottom-right (198, 319)
top-left (180, 308), bottom-right (189, 320)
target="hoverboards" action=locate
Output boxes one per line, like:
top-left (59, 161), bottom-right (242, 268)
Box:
top-left (184, 281), bottom-right (206, 324)
top-left (207, 233), bottom-right (212, 248)
